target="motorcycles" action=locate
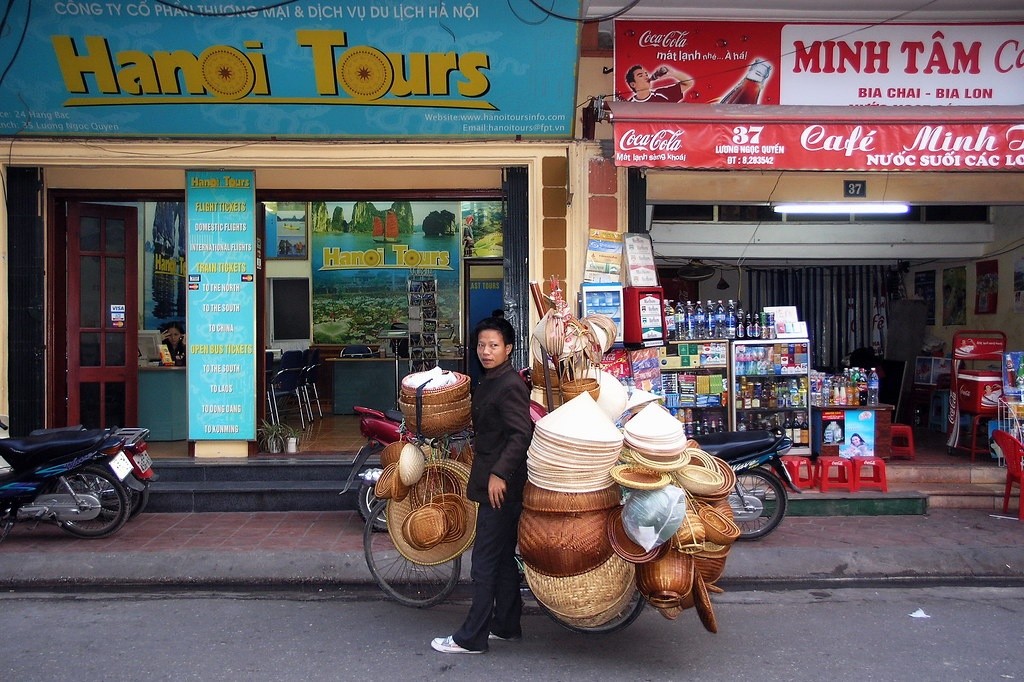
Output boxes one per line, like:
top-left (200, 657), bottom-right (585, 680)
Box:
top-left (684, 425), bottom-right (802, 541)
top-left (0, 419), bottom-right (159, 544)
top-left (338, 406), bottom-right (417, 532)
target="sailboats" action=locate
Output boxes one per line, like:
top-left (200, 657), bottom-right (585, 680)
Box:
top-left (372, 211), bottom-right (402, 243)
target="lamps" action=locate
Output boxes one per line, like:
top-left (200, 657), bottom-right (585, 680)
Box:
top-left (717, 269), bottom-right (729, 290)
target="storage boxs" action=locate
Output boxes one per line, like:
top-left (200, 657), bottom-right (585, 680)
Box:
top-left (914, 357), bottom-right (952, 385)
top-left (957, 369), bottom-right (1008, 414)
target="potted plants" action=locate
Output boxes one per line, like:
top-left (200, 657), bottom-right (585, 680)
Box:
top-left (257, 417), bottom-right (286, 455)
top-left (280, 423), bottom-right (309, 453)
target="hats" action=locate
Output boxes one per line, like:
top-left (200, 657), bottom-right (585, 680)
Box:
top-left (526, 389), bottom-right (689, 494)
top-left (533, 309), bottom-right (617, 367)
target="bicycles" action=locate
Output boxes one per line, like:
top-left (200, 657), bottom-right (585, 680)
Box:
top-left (363, 498), bottom-right (649, 636)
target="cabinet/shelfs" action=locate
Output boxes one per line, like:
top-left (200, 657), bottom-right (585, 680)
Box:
top-left (658, 337), bottom-right (811, 456)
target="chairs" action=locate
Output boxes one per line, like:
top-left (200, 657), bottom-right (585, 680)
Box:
top-left (991, 429), bottom-right (1024, 522)
top-left (265, 345), bottom-right (373, 431)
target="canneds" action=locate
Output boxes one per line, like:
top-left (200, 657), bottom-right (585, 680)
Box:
top-left (759, 311), bottom-right (775, 340)
top-left (739, 375), bottom-right (762, 398)
top-left (810, 377), bottom-right (823, 407)
top-left (671, 407), bottom-right (693, 434)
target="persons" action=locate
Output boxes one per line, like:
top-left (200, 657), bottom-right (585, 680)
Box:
top-left (159, 320), bottom-right (186, 366)
top-left (430, 317), bottom-right (535, 655)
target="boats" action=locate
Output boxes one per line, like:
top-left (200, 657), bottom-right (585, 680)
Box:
top-left (282, 226), bottom-right (301, 230)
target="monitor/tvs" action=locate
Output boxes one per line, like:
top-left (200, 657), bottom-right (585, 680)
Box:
top-left (138, 330), bottom-right (162, 360)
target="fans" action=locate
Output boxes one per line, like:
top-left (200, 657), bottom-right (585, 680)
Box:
top-left (676, 257), bottom-right (715, 281)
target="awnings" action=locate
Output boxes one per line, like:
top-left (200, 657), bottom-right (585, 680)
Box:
top-left (604, 101), bottom-right (1024, 174)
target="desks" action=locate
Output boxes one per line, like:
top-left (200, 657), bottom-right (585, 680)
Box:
top-left (928, 391), bottom-right (970, 434)
top-left (138, 365), bottom-right (186, 441)
top-left (326, 355), bottom-right (464, 415)
top-left (811, 403), bottom-right (895, 463)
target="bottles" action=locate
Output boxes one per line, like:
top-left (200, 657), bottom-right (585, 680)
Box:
top-left (718, 59), bottom-right (772, 104)
top-left (696, 418), bottom-right (724, 436)
top-left (736, 413), bottom-right (810, 446)
top-left (736, 376), bottom-right (808, 410)
top-left (663, 299), bottom-right (776, 339)
top-left (1015, 355), bottom-right (1024, 386)
top-left (811, 366), bottom-right (880, 407)
top-left (1006, 354), bottom-right (1017, 386)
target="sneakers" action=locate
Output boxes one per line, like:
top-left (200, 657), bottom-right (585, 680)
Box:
top-left (431, 636), bottom-right (486, 654)
top-left (488, 631), bottom-right (520, 642)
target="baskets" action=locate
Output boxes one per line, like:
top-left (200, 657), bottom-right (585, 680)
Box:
top-left (517, 450), bottom-right (740, 633)
top-left (374, 368), bottom-right (480, 566)
top-left (528, 367), bottom-right (600, 401)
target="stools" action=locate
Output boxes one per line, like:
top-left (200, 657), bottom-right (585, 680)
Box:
top-left (768, 456), bottom-right (814, 494)
top-left (812, 456), bottom-right (854, 494)
top-left (890, 423), bottom-right (915, 460)
top-left (849, 456), bottom-right (887, 494)
